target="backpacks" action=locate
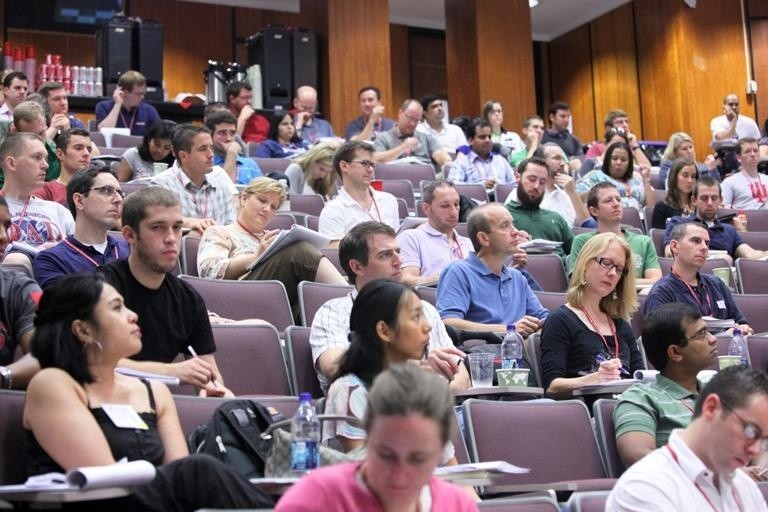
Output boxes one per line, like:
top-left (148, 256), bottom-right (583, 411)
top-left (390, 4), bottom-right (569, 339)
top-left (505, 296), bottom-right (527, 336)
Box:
top-left (204, 401), bottom-right (290, 480)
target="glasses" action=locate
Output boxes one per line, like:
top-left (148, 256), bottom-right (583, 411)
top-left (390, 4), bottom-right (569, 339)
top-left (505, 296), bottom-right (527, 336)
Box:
top-left (593, 257), bottom-right (624, 276)
top-left (721, 400), bottom-right (768, 452)
top-left (681, 329), bottom-right (705, 343)
top-left (90, 186), bottom-right (126, 199)
top-left (351, 159), bottom-right (376, 168)
top-left (610, 128), bottom-right (625, 135)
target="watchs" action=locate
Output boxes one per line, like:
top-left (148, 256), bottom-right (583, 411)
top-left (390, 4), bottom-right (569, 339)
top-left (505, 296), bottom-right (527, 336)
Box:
top-left (0, 366), bottom-right (15, 389)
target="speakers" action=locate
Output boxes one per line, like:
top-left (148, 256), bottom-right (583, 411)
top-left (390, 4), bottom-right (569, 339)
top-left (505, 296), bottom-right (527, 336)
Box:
top-left (243, 28), bottom-right (291, 111)
top-left (134, 17), bottom-right (166, 86)
top-left (291, 27), bottom-right (320, 111)
top-left (93, 17), bottom-right (134, 84)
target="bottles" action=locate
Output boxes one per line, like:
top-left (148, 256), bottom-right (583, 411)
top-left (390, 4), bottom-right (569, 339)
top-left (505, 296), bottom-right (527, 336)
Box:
top-left (737, 210), bottom-right (748, 232)
top-left (290, 391), bottom-right (322, 478)
top-left (728, 329), bottom-right (752, 367)
top-left (500, 324), bottom-right (525, 369)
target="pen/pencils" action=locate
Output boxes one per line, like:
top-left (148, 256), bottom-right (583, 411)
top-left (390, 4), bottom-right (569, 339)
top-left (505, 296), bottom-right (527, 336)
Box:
top-left (187, 345), bottom-right (217, 388)
top-left (448, 359), bottom-right (462, 386)
top-left (597, 355), bottom-right (630, 375)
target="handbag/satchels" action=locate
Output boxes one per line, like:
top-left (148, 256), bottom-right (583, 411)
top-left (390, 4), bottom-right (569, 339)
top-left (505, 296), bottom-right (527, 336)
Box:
top-left (264, 428), bottom-right (368, 480)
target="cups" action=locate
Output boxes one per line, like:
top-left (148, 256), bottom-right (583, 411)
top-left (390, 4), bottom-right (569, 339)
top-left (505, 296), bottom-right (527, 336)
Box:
top-left (371, 180), bottom-right (384, 191)
top-left (717, 355), bottom-right (742, 372)
top-left (496, 368), bottom-right (531, 387)
top-left (153, 162), bottom-right (169, 178)
top-left (467, 353), bottom-right (496, 388)
top-left (711, 267), bottom-right (729, 289)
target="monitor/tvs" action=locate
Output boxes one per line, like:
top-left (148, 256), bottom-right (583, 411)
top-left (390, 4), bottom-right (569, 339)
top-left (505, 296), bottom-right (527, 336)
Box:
top-left (5, 0), bottom-right (130, 39)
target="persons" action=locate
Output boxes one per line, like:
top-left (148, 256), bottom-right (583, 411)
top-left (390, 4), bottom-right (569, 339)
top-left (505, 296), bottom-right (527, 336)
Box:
top-left (94, 187), bottom-right (233, 396)
top-left (2, 71), bottom-right (768, 326)
top-left (21, 276), bottom-right (272, 510)
top-left (541, 233), bottom-right (645, 400)
top-left (0, 194), bottom-right (45, 390)
top-left (604, 365), bottom-right (768, 512)
top-left (612, 302), bottom-right (723, 468)
top-left (439, 202), bottom-right (548, 365)
top-left (645, 222), bottom-right (754, 339)
top-left (325, 280), bottom-right (460, 472)
top-left (309, 219), bottom-right (471, 398)
top-left (271, 364), bottom-right (480, 511)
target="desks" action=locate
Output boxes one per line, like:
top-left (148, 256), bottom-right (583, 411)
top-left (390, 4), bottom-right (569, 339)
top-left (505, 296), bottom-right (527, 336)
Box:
top-left (31, 93), bottom-right (110, 129)
